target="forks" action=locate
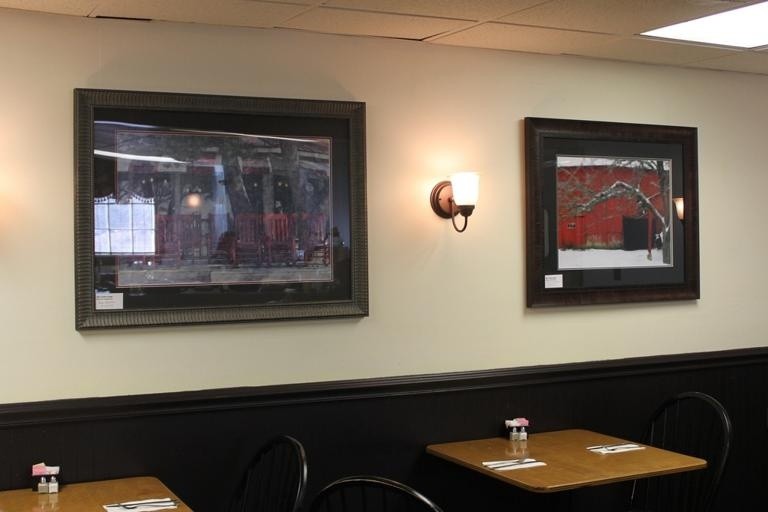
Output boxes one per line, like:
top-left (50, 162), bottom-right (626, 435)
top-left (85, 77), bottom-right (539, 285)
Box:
top-left (604, 446), bottom-right (642, 452)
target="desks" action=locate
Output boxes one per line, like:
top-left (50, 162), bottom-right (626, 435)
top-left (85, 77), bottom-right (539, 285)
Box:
top-left (425, 427), bottom-right (711, 511)
top-left (1, 475), bottom-right (196, 512)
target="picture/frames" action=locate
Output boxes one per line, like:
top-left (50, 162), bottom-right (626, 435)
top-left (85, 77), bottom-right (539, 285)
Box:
top-left (73, 87), bottom-right (369, 330)
top-left (525, 117), bottom-right (700, 310)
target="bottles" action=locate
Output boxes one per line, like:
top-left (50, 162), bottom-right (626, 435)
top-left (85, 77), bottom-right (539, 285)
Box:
top-left (509, 427), bottom-right (528, 440)
top-left (511, 441), bottom-right (528, 456)
top-left (37, 476), bottom-right (59, 494)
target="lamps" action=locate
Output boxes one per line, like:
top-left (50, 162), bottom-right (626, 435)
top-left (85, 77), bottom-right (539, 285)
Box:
top-left (673, 197), bottom-right (684, 227)
top-left (430, 170), bottom-right (481, 233)
top-left (181, 139), bottom-right (203, 209)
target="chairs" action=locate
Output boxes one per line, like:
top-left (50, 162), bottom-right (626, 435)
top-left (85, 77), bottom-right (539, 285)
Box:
top-left (127, 211), bottom-right (331, 268)
top-left (224, 434), bottom-right (309, 512)
top-left (308, 475), bottom-right (444, 512)
top-left (625, 391), bottom-right (735, 512)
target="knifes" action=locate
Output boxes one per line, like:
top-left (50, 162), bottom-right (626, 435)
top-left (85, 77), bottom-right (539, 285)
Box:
top-left (487, 461), bottom-right (546, 471)
top-left (106, 499), bottom-right (177, 507)
top-left (587, 442), bottom-right (634, 450)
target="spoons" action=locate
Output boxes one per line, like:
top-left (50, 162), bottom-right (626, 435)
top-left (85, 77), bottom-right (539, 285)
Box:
top-left (122, 502), bottom-right (176, 512)
